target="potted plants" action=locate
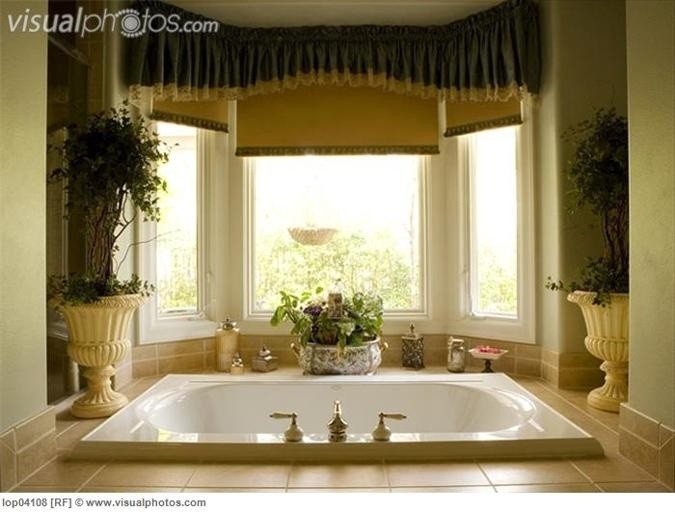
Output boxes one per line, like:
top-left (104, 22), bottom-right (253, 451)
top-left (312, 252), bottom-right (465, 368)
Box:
top-left (43, 94), bottom-right (185, 424)
top-left (271, 281), bottom-right (390, 375)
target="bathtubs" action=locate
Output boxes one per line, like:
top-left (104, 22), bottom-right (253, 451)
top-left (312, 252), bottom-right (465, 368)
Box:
top-left (79, 374), bottom-right (594, 471)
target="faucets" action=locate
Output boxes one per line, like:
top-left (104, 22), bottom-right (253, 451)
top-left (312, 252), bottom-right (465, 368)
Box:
top-left (326, 400), bottom-right (350, 442)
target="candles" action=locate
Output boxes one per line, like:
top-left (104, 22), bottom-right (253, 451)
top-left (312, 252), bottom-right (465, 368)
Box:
top-left (541, 77), bottom-right (630, 416)
